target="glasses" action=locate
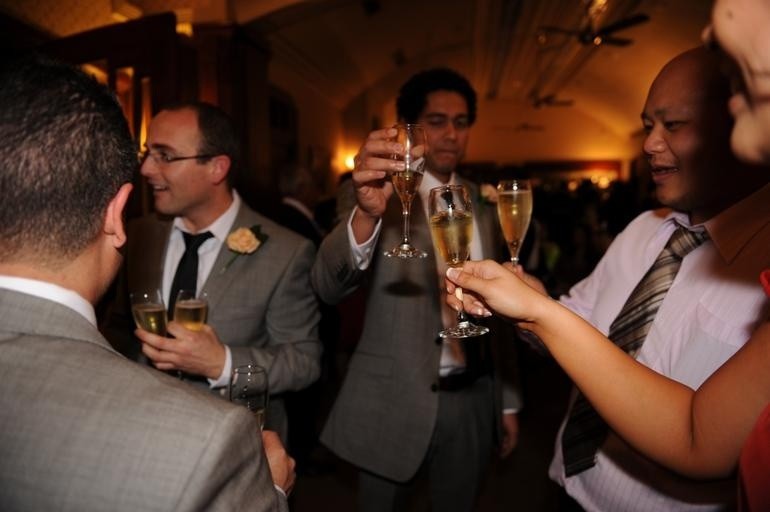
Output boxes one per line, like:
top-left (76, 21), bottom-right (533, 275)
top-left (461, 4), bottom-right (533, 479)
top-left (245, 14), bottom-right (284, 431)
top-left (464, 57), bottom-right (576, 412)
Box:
top-left (137, 147), bottom-right (217, 171)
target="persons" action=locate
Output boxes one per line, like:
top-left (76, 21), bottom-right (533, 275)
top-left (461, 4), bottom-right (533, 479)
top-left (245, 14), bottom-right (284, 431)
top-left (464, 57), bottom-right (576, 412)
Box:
top-left (94, 101), bottom-right (325, 457)
top-left (441, 1), bottom-right (770, 511)
top-left (0, 63), bottom-right (299, 512)
top-left (309, 64), bottom-right (527, 510)
top-left (274, 163), bottom-right (655, 486)
top-left (501, 44), bottom-right (770, 511)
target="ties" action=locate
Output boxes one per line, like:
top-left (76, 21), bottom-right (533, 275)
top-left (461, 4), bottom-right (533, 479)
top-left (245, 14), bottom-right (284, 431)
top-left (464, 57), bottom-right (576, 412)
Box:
top-left (153, 224), bottom-right (214, 371)
top-left (440, 187), bottom-right (491, 367)
top-left (559, 220), bottom-right (711, 478)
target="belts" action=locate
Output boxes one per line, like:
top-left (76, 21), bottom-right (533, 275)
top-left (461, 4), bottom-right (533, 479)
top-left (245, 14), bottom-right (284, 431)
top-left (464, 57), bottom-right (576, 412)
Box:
top-left (439, 363), bottom-right (487, 392)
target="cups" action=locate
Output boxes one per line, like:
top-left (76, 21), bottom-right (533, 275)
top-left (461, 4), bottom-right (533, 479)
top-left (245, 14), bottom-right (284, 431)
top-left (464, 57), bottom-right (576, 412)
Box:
top-left (127, 288), bottom-right (209, 351)
top-left (229, 366), bottom-right (269, 435)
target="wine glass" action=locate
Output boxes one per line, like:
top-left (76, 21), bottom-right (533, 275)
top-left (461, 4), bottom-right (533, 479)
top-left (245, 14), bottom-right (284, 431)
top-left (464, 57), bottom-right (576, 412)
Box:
top-left (383, 122), bottom-right (534, 339)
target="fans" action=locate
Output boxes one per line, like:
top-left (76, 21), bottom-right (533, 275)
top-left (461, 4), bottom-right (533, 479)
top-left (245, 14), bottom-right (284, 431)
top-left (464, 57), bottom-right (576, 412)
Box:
top-left (538, 12), bottom-right (650, 54)
